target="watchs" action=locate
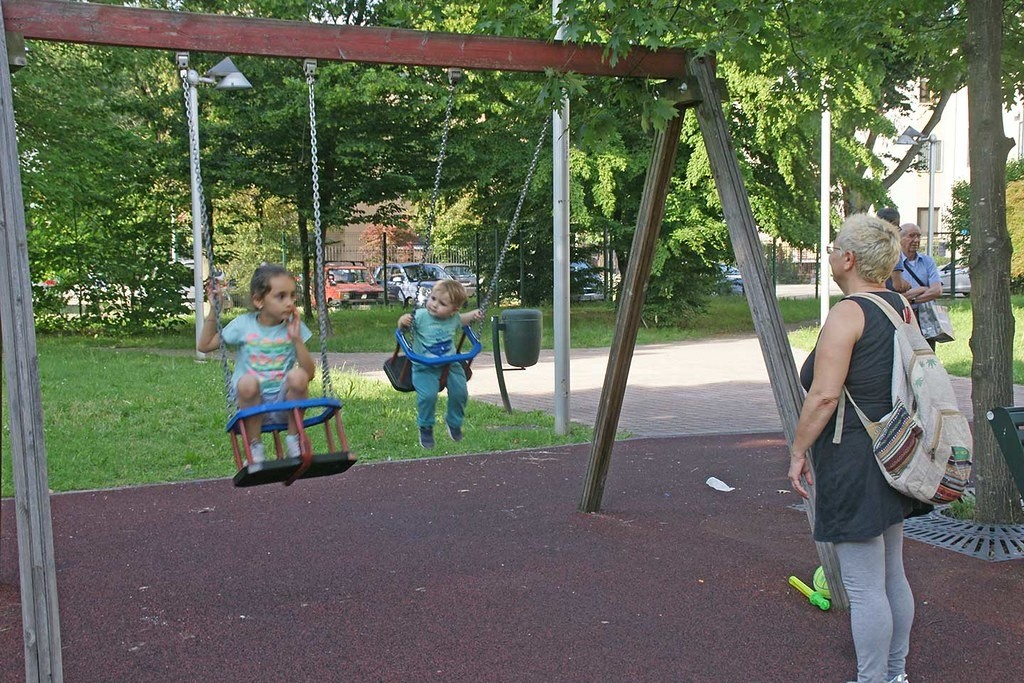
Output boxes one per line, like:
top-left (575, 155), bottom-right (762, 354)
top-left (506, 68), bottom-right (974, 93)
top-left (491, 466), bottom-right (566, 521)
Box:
top-left (911, 297), bottom-right (916, 303)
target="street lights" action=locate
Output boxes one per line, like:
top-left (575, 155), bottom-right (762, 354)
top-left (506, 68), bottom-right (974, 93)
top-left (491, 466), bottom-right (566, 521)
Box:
top-left (895, 133), bottom-right (937, 258)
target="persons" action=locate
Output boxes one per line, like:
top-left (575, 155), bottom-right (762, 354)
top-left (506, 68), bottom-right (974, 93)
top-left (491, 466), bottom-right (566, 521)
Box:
top-left (788, 214), bottom-right (935, 683)
top-left (875, 208), bottom-right (912, 295)
top-left (398, 279), bottom-right (484, 449)
top-left (198, 263), bottom-right (316, 467)
top-left (899, 223), bottom-right (945, 352)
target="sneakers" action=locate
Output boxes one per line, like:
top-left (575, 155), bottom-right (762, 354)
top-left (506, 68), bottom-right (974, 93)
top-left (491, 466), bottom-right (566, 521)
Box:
top-left (419, 426), bottom-right (435, 449)
top-left (447, 422), bottom-right (463, 442)
top-left (286, 434), bottom-right (303, 457)
top-left (243, 443), bottom-right (266, 466)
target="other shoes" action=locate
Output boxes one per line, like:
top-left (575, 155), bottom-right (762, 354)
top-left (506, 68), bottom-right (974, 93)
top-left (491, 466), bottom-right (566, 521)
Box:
top-left (890, 674), bottom-right (909, 683)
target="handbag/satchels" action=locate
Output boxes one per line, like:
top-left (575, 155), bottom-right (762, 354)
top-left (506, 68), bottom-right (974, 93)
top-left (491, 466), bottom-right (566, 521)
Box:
top-left (918, 299), bottom-right (956, 343)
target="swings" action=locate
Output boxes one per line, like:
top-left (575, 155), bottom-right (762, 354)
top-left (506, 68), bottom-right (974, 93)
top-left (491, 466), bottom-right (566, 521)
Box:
top-left (179, 61), bottom-right (359, 489)
top-left (381, 81), bottom-right (554, 393)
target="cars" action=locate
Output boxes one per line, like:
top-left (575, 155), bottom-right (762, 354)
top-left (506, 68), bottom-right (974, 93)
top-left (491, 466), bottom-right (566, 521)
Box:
top-left (433, 262), bottom-right (482, 288)
top-left (569, 259), bottom-right (606, 303)
top-left (372, 261), bottom-right (453, 309)
top-left (938, 256), bottom-right (972, 297)
top-left (308, 261), bottom-right (397, 309)
top-left (708, 263), bottom-right (745, 297)
top-left (33, 257), bottom-right (237, 312)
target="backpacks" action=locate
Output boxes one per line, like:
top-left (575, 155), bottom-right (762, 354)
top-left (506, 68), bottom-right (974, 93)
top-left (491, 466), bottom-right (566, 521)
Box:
top-left (833, 292), bottom-right (973, 505)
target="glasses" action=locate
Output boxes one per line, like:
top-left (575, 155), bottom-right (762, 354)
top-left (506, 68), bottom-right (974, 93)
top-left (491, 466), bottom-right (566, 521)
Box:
top-left (895, 223), bottom-right (902, 232)
top-left (825, 240), bottom-right (841, 254)
